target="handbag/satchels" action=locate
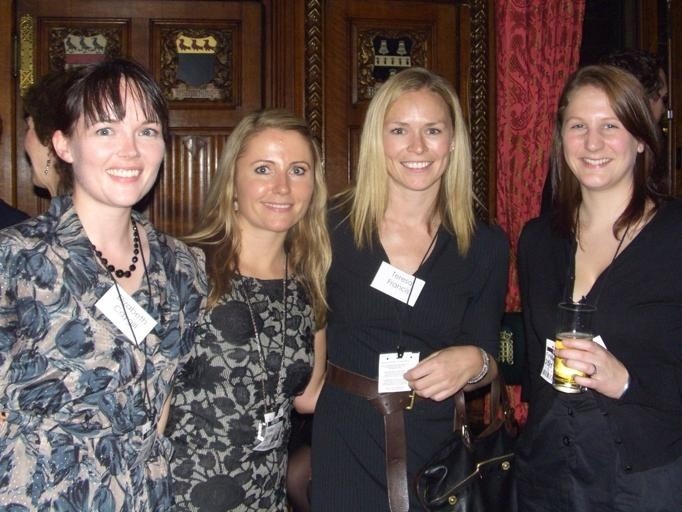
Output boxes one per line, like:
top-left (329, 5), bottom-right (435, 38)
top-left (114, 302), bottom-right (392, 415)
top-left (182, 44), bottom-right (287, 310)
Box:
top-left (409, 377), bottom-right (522, 512)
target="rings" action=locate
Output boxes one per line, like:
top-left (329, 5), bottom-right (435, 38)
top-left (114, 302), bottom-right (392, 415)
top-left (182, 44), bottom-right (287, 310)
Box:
top-left (588, 365), bottom-right (598, 378)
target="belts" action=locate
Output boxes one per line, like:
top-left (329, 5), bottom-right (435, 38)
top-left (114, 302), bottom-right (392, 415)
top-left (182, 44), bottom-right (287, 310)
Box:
top-left (325, 359), bottom-right (426, 512)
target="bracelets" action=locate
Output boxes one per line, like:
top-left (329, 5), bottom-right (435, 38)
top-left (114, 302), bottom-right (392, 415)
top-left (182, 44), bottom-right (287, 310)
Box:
top-left (468, 347), bottom-right (490, 385)
top-left (619, 371), bottom-right (630, 398)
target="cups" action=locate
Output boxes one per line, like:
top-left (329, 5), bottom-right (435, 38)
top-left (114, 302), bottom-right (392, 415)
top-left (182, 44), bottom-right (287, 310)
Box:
top-left (551, 301), bottom-right (597, 395)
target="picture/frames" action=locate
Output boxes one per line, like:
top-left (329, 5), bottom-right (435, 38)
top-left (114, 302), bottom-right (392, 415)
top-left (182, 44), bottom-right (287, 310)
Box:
top-left (343, 13), bottom-right (442, 113)
top-left (35, 14), bottom-right (134, 86)
top-left (147, 15), bottom-right (244, 112)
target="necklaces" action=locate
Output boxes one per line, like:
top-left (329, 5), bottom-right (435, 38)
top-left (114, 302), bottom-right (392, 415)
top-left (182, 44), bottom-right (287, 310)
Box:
top-left (91, 214), bottom-right (140, 277)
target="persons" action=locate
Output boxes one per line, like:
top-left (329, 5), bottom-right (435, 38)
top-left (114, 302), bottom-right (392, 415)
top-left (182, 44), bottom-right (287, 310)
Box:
top-left (165, 109), bottom-right (334, 511)
top-left (1, 55), bottom-right (200, 512)
top-left (512, 63), bottom-right (682, 511)
top-left (22, 74), bottom-right (74, 196)
top-left (285, 65), bottom-right (511, 510)
